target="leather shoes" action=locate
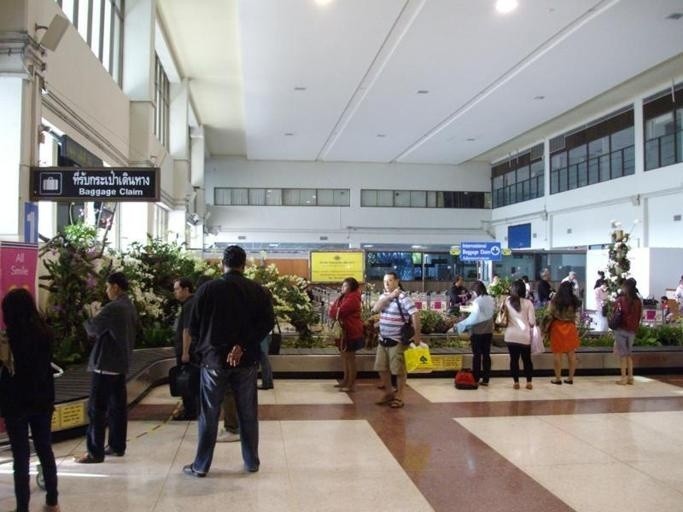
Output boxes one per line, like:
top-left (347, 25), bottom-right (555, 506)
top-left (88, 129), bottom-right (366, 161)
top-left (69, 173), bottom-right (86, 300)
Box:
top-left (42, 503), bottom-right (61, 511)
top-left (550, 379), bottom-right (561, 384)
top-left (172, 410), bottom-right (199, 421)
top-left (247, 456), bottom-right (260, 472)
top-left (103, 443), bottom-right (124, 456)
top-left (182, 462), bottom-right (206, 478)
top-left (563, 378), bottom-right (573, 384)
top-left (74, 452), bottom-right (104, 463)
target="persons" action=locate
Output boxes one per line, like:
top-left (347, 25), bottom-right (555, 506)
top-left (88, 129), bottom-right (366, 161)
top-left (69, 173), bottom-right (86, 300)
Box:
top-left (72, 272), bottom-right (138, 466)
top-left (1, 287), bottom-right (60, 511)
top-left (327, 272), bottom-right (421, 410)
top-left (591, 268), bottom-right (683, 385)
top-left (168, 244), bottom-right (273, 478)
top-left (446, 266), bottom-right (581, 388)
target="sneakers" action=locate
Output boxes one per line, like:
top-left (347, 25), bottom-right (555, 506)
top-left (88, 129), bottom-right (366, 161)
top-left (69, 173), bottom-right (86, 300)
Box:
top-left (257, 383), bottom-right (274, 390)
top-left (526, 383), bottom-right (532, 389)
top-left (615, 378), bottom-right (635, 385)
top-left (216, 430), bottom-right (240, 442)
top-left (480, 382), bottom-right (488, 386)
top-left (513, 383), bottom-right (520, 389)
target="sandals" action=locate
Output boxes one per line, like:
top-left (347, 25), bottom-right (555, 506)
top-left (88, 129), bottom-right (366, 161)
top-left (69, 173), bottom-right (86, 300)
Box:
top-left (375, 393), bottom-right (393, 405)
top-left (390, 397), bottom-right (405, 408)
top-left (334, 382), bottom-right (357, 392)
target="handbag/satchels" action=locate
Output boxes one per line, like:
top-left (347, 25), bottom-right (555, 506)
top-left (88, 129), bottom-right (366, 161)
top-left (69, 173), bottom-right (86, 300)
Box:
top-left (455, 366), bottom-right (479, 389)
top-left (268, 332), bottom-right (282, 355)
top-left (169, 360), bottom-right (195, 397)
top-left (403, 339), bottom-right (433, 374)
top-left (326, 307), bottom-right (343, 340)
top-left (606, 306), bottom-right (623, 330)
top-left (539, 310), bottom-right (554, 333)
top-left (379, 337), bottom-right (398, 348)
top-left (530, 319), bottom-right (546, 356)
top-left (495, 297), bottom-right (509, 327)
top-left (399, 322), bottom-right (415, 345)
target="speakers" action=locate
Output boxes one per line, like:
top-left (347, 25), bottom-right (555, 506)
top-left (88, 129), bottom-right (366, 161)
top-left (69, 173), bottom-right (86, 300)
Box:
top-left (39, 12), bottom-right (75, 52)
